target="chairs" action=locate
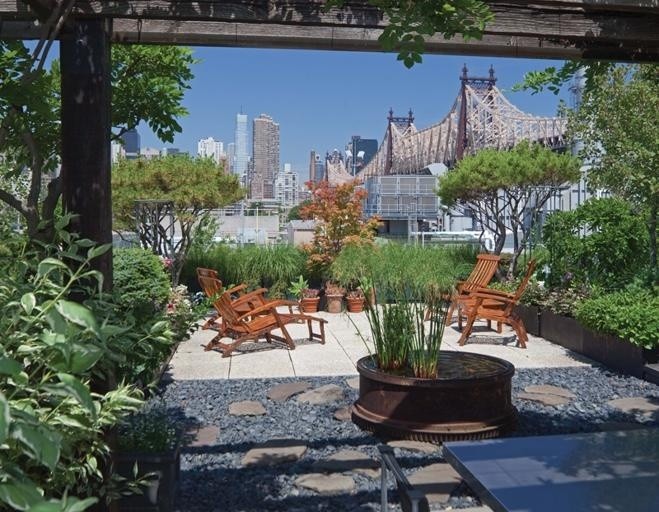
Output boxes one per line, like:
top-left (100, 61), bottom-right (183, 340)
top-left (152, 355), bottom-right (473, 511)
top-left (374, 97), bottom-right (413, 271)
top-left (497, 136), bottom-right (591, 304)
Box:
top-left (196, 276), bottom-right (328, 358)
top-left (459, 257), bottom-right (536, 348)
top-left (196, 267), bottom-right (275, 339)
top-left (425, 254), bottom-right (500, 327)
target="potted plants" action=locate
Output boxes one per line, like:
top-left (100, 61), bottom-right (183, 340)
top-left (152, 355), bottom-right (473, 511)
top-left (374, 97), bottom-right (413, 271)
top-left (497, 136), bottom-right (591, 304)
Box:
top-left (346, 288), bottom-right (365, 312)
top-left (324, 280), bottom-right (345, 313)
top-left (300, 288), bottom-right (318, 312)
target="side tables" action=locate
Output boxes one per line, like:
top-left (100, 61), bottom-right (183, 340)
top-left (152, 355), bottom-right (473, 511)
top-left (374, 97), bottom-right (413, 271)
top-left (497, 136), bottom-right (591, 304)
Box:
top-left (458, 299), bottom-right (506, 332)
top-left (234, 304), bottom-right (273, 344)
